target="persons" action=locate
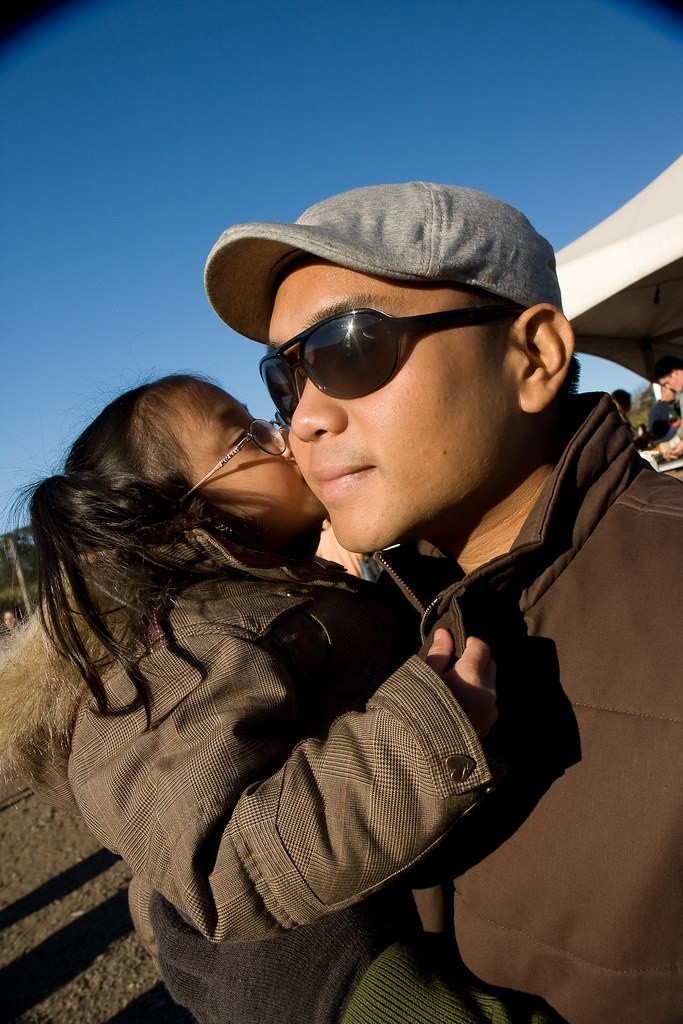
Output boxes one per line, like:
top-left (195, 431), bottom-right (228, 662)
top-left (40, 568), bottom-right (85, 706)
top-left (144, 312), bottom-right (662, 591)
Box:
top-left (200, 182), bottom-right (683, 1024)
top-left (611, 354), bottom-right (683, 470)
top-left (20, 371), bottom-right (555, 1024)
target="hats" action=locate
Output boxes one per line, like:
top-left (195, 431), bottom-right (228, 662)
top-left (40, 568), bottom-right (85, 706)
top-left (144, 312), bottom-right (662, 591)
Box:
top-left (202, 180), bottom-right (565, 346)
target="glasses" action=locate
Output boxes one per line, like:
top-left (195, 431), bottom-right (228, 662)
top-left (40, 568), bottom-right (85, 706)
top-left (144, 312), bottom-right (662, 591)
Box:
top-left (176, 419), bottom-right (288, 508)
top-left (256, 299), bottom-right (516, 424)
